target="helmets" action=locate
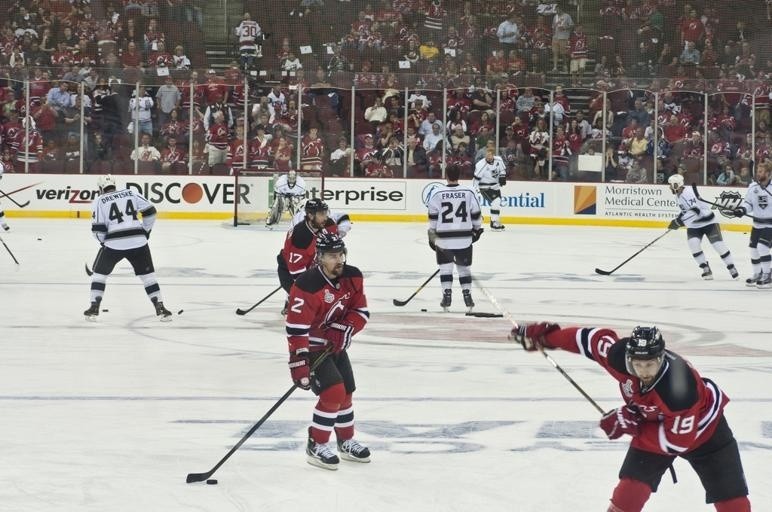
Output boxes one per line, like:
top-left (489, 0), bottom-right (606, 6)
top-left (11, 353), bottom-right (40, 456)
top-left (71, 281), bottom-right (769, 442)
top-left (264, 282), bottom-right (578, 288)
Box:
top-left (668, 174), bottom-right (684, 194)
top-left (289, 170), bottom-right (297, 183)
top-left (317, 233), bottom-right (345, 252)
top-left (306, 198), bottom-right (330, 229)
top-left (625, 326), bottom-right (665, 380)
top-left (98, 174), bottom-right (117, 192)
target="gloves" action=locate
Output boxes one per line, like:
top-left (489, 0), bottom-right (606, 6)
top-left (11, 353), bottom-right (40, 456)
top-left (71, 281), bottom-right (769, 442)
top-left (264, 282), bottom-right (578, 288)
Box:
top-left (500, 177), bottom-right (505, 186)
top-left (601, 406), bottom-right (638, 439)
top-left (429, 230), bottom-right (439, 250)
top-left (736, 208), bottom-right (742, 217)
top-left (672, 220), bottom-right (682, 230)
top-left (292, 359), bottom-right (313, 390)
top-left (507, 322), bottom-right (534, 351)
top-left (472, 227), bottom-right (483, 241)
top-left (327, 322), bottom-right (353, 355)
top-left (668, 223), bottom-right (678, 230)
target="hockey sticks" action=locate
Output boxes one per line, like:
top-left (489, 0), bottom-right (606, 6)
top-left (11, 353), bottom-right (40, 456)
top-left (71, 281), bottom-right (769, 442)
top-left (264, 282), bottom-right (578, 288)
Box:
top-left (186, 346), bottom-right (334, 484)
top-left (0, 190), bottom-right (32, 208)
top-left (690, 182), bottom-right (753, 218)
top-left (595, 229), bottom-right (671, 276)
top-left (392, 229), bottom-right (483, 307)
top-left (84, 257), bottom-right (125, 277)
top-left (235, 286), bottom-right (282, 315)
top-left (469, 276), bottom-right (608, 416)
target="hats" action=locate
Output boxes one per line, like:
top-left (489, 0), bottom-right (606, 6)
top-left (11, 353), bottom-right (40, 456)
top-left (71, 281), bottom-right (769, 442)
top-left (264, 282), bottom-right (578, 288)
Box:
top-left (338, 136), bottom-right (347, 141)
top-left (366, 134), bottom-right (372, 137)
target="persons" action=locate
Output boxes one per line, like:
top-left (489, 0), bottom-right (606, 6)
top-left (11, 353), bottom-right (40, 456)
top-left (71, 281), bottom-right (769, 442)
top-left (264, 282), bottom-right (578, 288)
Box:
top-left (428, 164), bottom-right (484, 307)
top-left (286, 199), bottom-right (352, 239)
top-left (447, 1), bottom-right (504, 162)
top-left (472, 148), bottom-right (506, 233)
top-left (266, 169), bottom-right (307, 225)
top-left (668, 174), bottom-right (740, 282)
top-left (0, 162), bottom-right (11, 232)
top-left (277, 198), bottom-right (337, 315)
top-left (733, 162), bottom-right (772, 291)
top-left (511, 322), bottom-right (752, 512)
top-left (284, 233), bottom-right (373, 472)
top-left (84, 174), bottom-right (173, 323)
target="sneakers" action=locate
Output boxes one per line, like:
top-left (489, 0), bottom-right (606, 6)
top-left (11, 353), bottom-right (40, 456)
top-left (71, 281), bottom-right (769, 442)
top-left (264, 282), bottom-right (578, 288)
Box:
top-left (462, 289), bottom-right (474, 307)
top-left (441, 289), bottom-right (452, 307)
top-left (727, 264), bottom-right (738, 277)
top-left (490, 220), bottom-right (505, 229)
top-left (156, 302), bottom-right (172, 317)
top-left (338, 439), bottom-right (370, 457)
top-left (306, 439), bottom-right (338, 464)
top-left (84, 302), bottom-right (99, 316)
top-left (755, 268), bottom-right (771, 285)
top-left (700, 261), bottom-right (710, 277)
top-left (5, 227), bottom-right (9, 231)
top-left (747, 270), bottom-right (762, 283)
